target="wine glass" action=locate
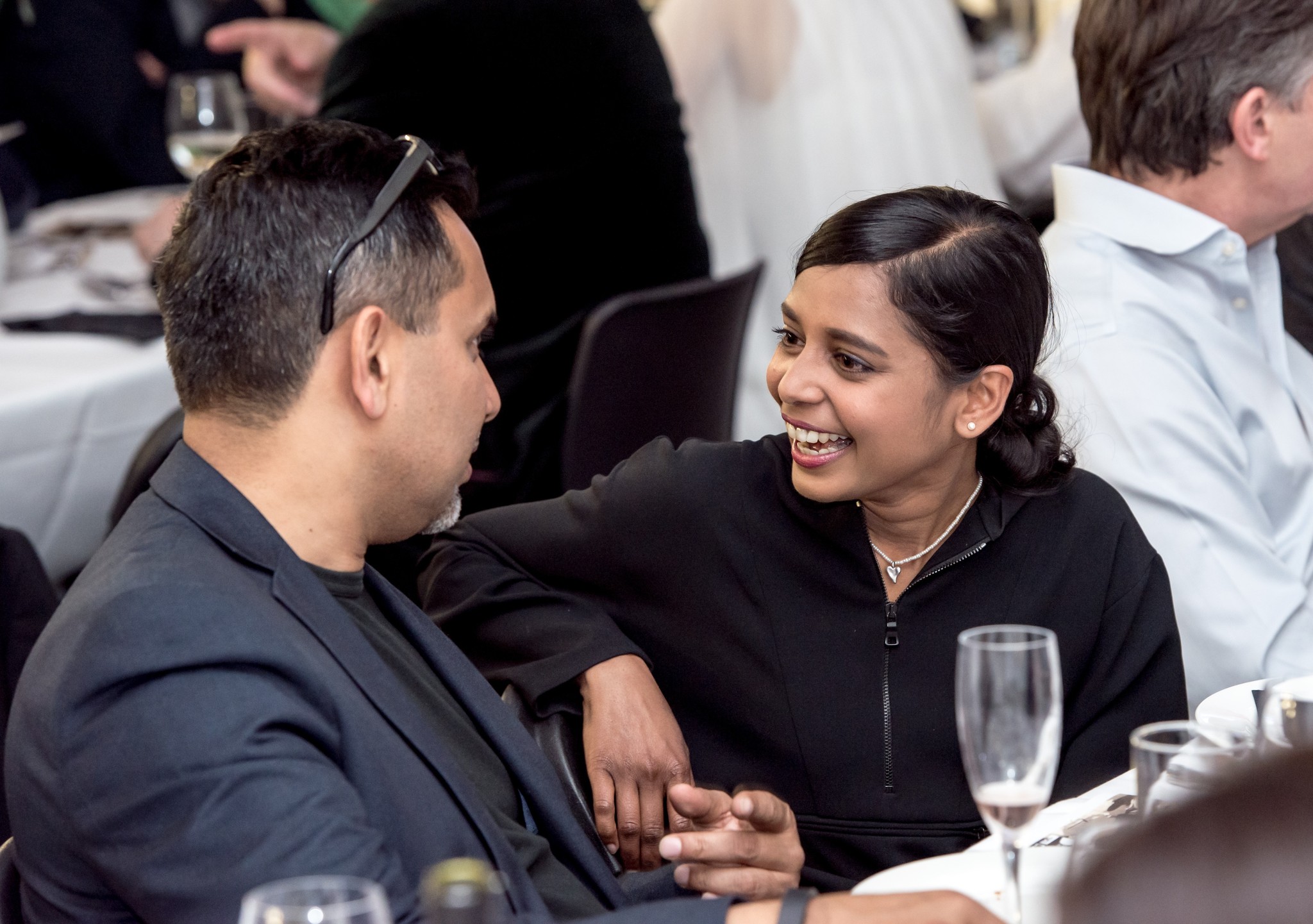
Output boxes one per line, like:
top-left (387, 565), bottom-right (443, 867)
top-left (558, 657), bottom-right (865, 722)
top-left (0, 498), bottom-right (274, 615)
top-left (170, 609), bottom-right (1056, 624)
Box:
top-left (955, 626), bottom-right (1063, 924)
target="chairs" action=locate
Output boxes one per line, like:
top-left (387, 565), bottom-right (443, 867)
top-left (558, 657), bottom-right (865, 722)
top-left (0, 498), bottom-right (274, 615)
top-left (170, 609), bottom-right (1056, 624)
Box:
top-left (463, 264), bottom-right (768, 876)
top-left (0, 412), bottom-right (186, 924)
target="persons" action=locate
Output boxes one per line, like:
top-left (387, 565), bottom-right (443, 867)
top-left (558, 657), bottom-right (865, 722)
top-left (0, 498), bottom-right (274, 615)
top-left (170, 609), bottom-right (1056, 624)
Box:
top-left (422, 188), bottom-right (1185, 894)
top-left (3, 122), bottom-right (1006, 924)
top-left (0, 0), bottom-right (1313, 721)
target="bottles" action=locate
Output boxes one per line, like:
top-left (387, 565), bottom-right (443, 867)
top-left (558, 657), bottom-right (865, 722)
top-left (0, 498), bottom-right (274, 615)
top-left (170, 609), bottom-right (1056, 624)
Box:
top-left (414, 857), bottom-right (499, 924)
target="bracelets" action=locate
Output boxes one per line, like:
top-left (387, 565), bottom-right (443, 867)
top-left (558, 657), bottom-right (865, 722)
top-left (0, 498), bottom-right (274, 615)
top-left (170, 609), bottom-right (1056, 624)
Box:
top-left (777, 888), bottom-right (818, 924)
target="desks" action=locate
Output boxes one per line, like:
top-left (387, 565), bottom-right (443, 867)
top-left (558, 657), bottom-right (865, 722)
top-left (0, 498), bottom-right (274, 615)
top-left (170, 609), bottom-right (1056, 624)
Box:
top-left (957, 770), bottom-right (1136, 924)
top-left (0, 187), bottom-right (198, 584)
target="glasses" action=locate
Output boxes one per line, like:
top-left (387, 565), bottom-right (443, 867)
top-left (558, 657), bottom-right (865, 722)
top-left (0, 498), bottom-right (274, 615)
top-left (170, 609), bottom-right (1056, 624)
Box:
top-left (320, 135), bottom-right (444, 334)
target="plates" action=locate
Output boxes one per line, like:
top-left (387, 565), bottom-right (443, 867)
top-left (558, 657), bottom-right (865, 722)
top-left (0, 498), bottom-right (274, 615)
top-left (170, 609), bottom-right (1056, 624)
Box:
top-left (1194, 678), bottom-right (1292, 749)
top-left (849, 847), bottom-right (1074, 924)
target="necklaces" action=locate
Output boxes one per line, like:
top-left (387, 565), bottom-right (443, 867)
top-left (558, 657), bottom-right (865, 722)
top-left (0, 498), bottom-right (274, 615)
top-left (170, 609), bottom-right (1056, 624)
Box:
top-left (871, 472), bottom-right (982, 585)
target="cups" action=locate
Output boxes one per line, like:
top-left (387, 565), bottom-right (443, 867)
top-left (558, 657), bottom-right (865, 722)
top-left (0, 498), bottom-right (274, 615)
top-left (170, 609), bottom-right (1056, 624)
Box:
top-left (1129, 721), bottom-right (1196, 822)
top-left (167, 70), bottom-right (250, 181)
top-left (237, 874), bottom-right (394, 924)
